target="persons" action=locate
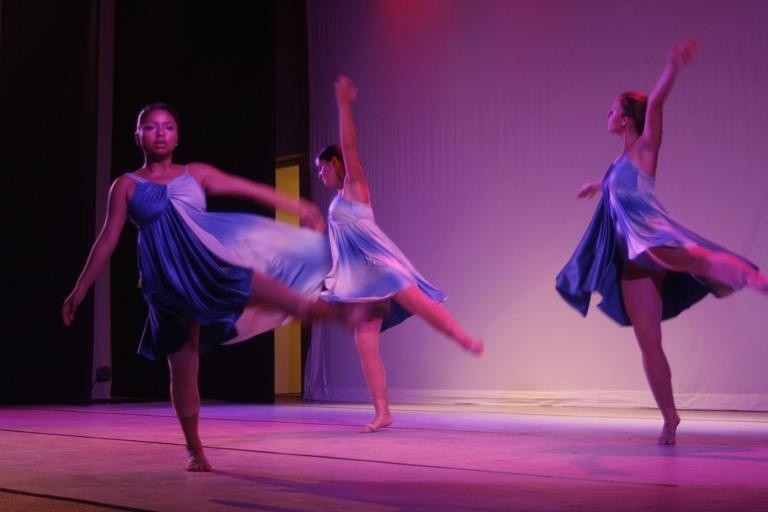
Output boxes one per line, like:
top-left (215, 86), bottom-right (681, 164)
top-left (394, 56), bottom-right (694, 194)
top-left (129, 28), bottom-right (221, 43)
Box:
top-left (314, 72), bottom-right (483, 433)
top-left (555, 34), bottom-right (768, 444)
top-left (61, 102), bottom-right (390, 472)
